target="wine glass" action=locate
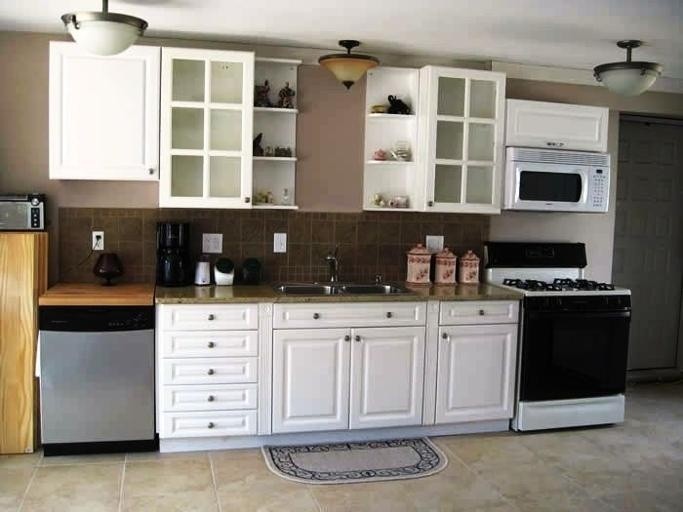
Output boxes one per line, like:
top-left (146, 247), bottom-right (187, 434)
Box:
top-left (92, 252), bottom-right (122, 287)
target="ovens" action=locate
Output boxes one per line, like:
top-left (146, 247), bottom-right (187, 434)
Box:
top-left (514, 309), bottom-right (632, 433)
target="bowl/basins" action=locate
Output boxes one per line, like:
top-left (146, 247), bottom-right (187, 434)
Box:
top-left (370, 105), bottom-right (387, 113)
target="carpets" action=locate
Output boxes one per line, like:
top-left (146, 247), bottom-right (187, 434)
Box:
top-left (260, 433), bottom-right (448, 484)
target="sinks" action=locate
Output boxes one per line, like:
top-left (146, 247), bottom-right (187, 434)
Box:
top-left (280, 286), bottom-right (344, 294)
top-left (345, 285), bottom-right (404, 294)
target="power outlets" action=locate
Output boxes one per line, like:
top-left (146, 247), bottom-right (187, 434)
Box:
top-left (91, 229), bottom-right (103, 251)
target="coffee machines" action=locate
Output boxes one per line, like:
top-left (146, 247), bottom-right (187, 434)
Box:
top-left (155, 220), bottom-right (190, 286)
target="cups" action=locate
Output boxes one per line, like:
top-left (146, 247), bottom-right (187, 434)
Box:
top-left (389, 195), bottom-right (407, 208)
top-left (396, 141), bottom-right (408, 159)
top-left (193, 261), bottom-right (210, 286)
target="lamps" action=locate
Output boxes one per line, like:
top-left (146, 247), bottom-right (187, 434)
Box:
top-left (316, 39), bottom-right (381, 89)
top-left (592, 38), bottom-right (663, 101)
top-left (59, 0), bottom-right (147, 56)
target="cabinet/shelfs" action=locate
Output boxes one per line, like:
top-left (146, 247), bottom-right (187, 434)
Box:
top-left (501, 96), bottom-right (611, 155)
top-left (252, 58), bottom-right (303, 209)
top-left (158, 48), bottom-right (256, 209)
top-left (157, 301), bottom-right (261, 453)
top-left (48, 41), bottom-right (158, 183)
top-left (423, 66), bottom-right (510, 218)
top-left (363, 66), bottom-right (423, 215)
top-left (431, 301), bottom-right (521, 437)
top-left (265, 302), bottom-right (430, 434)
top-left (0, 227), bottom-right (48, 457)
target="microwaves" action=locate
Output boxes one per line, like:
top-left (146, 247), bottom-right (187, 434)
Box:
top-left (503, 148), bottom-right (611, 214)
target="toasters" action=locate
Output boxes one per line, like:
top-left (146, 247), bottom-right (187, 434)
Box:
top-left (0, 193), bottom-right (48, 232)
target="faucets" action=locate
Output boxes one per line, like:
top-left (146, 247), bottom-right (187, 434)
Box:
top-left (324, 254), bottom-right (338, 283)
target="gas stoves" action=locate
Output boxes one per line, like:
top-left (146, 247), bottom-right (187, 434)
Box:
top-left (494, 276), bottom-right (632, 312)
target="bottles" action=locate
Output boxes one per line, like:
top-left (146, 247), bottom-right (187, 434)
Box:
top-left (409, 284), bottom-right (481, 297)
top-left (213, 258), bottom-right (234, 286)
top-left (238, 258), bottom-right (261, 285)
top-left (404, 241), bottom-right (480, 286)
